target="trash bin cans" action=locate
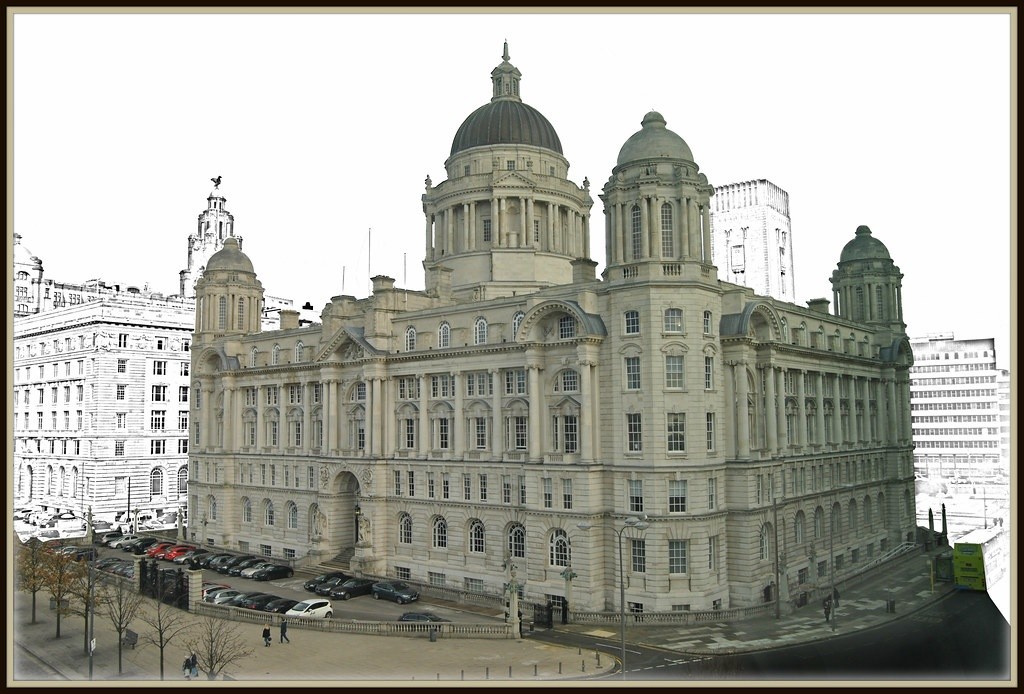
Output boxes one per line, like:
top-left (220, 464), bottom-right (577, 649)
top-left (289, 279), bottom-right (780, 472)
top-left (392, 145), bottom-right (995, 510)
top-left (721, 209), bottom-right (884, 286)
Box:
top-left (50, 598), bottom-right (56, 610)
top-left (61, 598), bottom-right (69, 613)
top-left (430, 628), bottom-right (437, 642)
top-left (886, 600), bottom-right (895, 613)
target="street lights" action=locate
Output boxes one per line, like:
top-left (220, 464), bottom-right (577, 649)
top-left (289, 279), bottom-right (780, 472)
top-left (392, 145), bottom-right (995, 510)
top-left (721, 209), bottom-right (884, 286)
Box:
top-left (576, 515), bottom-right (650, 680)
top-left (973, 485), bottom-right (986, 528)
top-left (773, 496), bottom-right (788, 618)
top-left (122, 475), bottom-right (131, 521)
top-left (828, 483), bottom-right (854, 629)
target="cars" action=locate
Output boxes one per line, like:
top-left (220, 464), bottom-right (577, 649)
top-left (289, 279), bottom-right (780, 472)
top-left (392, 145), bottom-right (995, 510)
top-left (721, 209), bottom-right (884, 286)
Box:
top-left (914, 473), bottom-right (1009, 485)
top-left (82, 520), bottom-right (114, 531)
top-left (156, 511), bottom-right (178, 524)
top-left (108, 534), bottom-right (295, 581)
top-left (89, 556), bottom-right (176, 583)
top-left (201, 580), bottom-right (299, 614)
top-left (174, 518), bottom-right (187, 527)
top-left (13, 507), bottom-right (75, 528)
top-left (132, 521), bottom-right (164, 532)
top-left (45, 542), bottom-right (78, 559)
top-left (371, 581), bottom-right (420, 604)
top-left (303, 572), bottom-right (380, 601)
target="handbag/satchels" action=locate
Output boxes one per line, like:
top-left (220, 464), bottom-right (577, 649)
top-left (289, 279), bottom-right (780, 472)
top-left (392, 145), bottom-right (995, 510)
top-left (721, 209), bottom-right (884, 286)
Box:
top-left (191, 666), bottom-right (197, 674)
top-left (268, 636), bottom-right (272, 641)
top-left (185, 667), bottom-right (190, 677)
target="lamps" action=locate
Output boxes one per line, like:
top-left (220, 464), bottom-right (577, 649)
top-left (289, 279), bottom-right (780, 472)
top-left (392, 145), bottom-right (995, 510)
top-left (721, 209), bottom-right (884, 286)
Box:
top-left (354, 506), bottom-right (361, 515)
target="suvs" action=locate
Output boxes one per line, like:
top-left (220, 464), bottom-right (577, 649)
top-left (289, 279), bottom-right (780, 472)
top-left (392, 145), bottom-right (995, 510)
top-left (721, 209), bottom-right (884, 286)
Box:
top-left (67, 548), bottom-right (98, 562)
top-left (96, 532), bottom-right (123, 547)
top-left (285, 597), bottom-right (333, 624)
top-left (396, 611), bottom-right (453, 632)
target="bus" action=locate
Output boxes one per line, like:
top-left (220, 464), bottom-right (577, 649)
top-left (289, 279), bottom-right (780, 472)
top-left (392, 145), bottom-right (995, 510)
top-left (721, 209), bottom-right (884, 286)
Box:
top-left (954, 525), bottom-right (1013, 591)
top-left (120, 512), bottom-right (153, 526)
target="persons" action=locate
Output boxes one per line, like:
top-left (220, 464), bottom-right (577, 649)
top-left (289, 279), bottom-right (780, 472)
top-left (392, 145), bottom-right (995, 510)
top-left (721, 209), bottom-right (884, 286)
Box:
top-left (262, 623), bottom-right (271, 647)
top-left (182, 651), bottom-right (199, 680)
top-left (824, 601), bottom-right (831, 621)
top-left (279, 618), bottom-right (290, 644)
top-left (833, 588), bottom-right (840, 608)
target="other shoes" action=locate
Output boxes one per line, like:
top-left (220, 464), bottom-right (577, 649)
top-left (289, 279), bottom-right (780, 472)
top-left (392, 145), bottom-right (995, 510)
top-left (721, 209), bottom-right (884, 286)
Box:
top-left (288, 640), bottom-right (289, 642)
top-left (265, 645), bottom-right (267, 646)
top-left (269, 643), bottom-right (270, 646)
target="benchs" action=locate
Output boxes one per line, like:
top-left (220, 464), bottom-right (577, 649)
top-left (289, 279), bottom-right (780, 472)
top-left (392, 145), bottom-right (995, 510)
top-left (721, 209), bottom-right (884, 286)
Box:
top-left (121, 628), bottom-right (139, 649)
